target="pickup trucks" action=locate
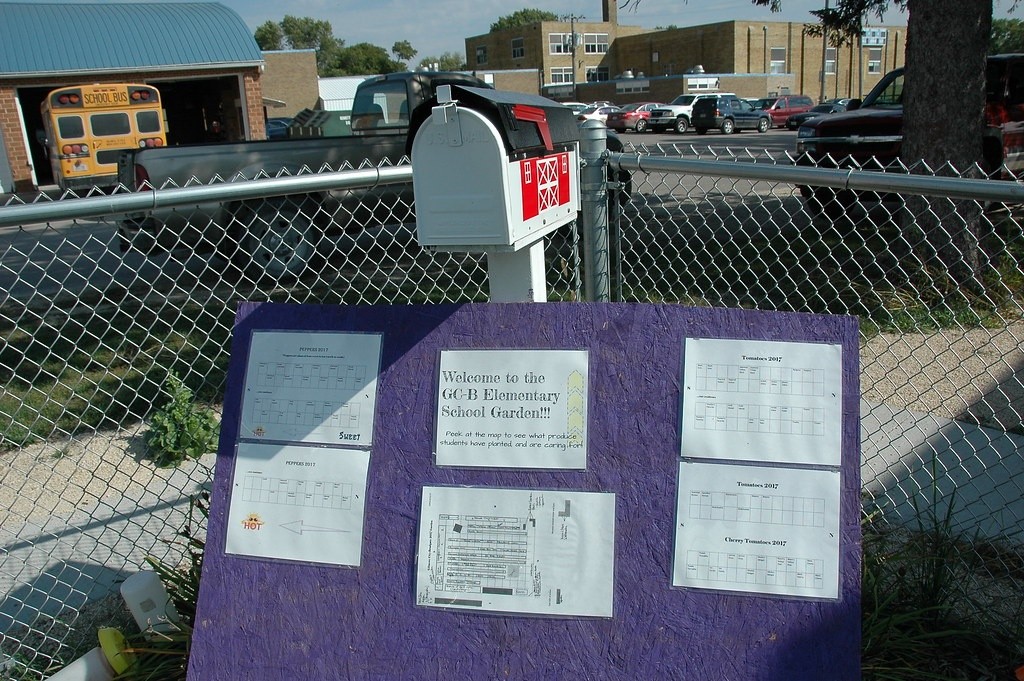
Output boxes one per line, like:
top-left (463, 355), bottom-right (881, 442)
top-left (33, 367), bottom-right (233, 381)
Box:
top-left (115, 70), bottom-right (632, 280)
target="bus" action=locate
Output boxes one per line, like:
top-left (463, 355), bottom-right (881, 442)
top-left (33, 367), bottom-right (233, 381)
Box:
top-left (38, 83), bottom-right (167, 199)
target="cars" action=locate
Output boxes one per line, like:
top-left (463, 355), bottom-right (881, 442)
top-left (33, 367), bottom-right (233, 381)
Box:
top-left (786, 103), bottom-right (849, 130)
top-left (605, 101), bottom-right (668, 133)
top-left (559, 101), bottom-right (587, 115)
top-left (265, 116), bottom-right (295, 139)
top-left (574, 105), bottom-right (621, 126)
top-left (824, 98), bottom-right (853, 107)
top-left (740, 98), bottom-right (763, 110)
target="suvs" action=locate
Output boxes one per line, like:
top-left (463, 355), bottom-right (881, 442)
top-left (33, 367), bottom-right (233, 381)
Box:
top-left (690, 94), bottom-right (772, 135)
top-left (646, 91), bottom-right (738, 134)
top-left (787, 52), bottom-right (1024, 223)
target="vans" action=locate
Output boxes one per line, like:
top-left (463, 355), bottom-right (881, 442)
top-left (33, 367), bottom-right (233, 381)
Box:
top-left (753, 95), bottom-right (814, 129)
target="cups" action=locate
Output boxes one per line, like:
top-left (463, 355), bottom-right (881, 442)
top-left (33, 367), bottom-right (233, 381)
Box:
top-left (120, 570), bottom-right (180, 641)
